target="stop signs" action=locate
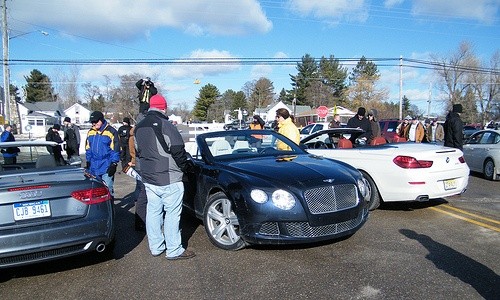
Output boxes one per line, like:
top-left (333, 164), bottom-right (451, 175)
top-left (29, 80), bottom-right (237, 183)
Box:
top-left (317, 106), bottom-right (329, 118)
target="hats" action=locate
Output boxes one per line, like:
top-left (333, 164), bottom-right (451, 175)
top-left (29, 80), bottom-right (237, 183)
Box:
top-left (150, 95), bottom-right (167, 110)
top-left (358, 107), bottom-right (365, 116)
top-left (54, 124), bottom-right (60, 130)
top-left (65, 117), bottom-right (71, 122)
top-left (369, 109), bottom-right (378, 122)
top-left (412, 116), bottom-right (417, 120)
top-left (89, 111), bottom-right (102, 121)
top-left (453, 104), bottom-right (465, 113)
top-left (123, 117), bottom-right (129, 122)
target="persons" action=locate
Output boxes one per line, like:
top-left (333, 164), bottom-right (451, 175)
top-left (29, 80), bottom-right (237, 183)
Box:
top-left (46, 124), bottom-right (64, 154)
top-left (271, 120), bottom-right (279, 147)
top-left (0, 124), bottom-right (20, 164)
top-left (245, 115), bottom-right (265, 148)
top-left (366, 109), bottom-right (381, 144)
top-left (128, 117), bottom-right (148, 224)
top-left (487, 121), bottom-right (498, 130)
top-left (328, 114), bottom-right (343, 143)
top-left (396, 119), bottom-right (409, 142)
top-left (63, 117), bottom-right (80, 159)
top-left (85, 111), bottom-right (119, 194)
top-left (118, 117), bottom-right (133, 174)
top-left (276, 108), bottom-right (300, 151)
top-left (404, 116), bottom-right (424, 143)
top-left (444, 104), bottom-right (463, 151)
top-left (135, 94), bottom-right (195, 259)
top-left (135, 77), bottom-right (158, 113)
top-left (346, 107), bottom-right (372, 144)
top-left (423, 119), bottom-right (444, 145)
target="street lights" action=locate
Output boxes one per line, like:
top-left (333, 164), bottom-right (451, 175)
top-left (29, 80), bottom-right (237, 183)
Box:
top-left (3, 29), bottom-right (49, 125)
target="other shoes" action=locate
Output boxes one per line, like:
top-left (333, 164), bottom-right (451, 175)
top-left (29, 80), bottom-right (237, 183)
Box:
top-left (135, 225), bottom-right (146, 232)
top-left (168, 250), bottom-right (196, 260)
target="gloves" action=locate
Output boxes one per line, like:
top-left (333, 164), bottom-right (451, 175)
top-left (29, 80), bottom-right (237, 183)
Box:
top-left (108, 162), bottom-right (117, 177)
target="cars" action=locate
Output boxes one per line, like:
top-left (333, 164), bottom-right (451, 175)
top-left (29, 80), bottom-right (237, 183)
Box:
top-left (462, 128), bottom-right (500, 182)
top-left (78, 119), bottom-right (500, 166)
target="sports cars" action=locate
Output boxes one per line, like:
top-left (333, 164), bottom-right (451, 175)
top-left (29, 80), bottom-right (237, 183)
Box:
top-left (299, 127), bottom-right (471, 212)
top-left (182, 129), bottom-right (370, 252)
top-left (0, 140), bottom-right (116, 268)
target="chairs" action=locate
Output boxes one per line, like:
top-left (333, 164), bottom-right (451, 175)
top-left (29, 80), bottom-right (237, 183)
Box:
top-left (210, 139), bottom-right (233, 154)
top-left (338, 138), bottom-right (353, 149)
top-left (36, 154), bottom-right (57, 167)
top-left (371, 136), bottom-right (386, 145)
top-left (232, 140), bottom-right (250, 150)
